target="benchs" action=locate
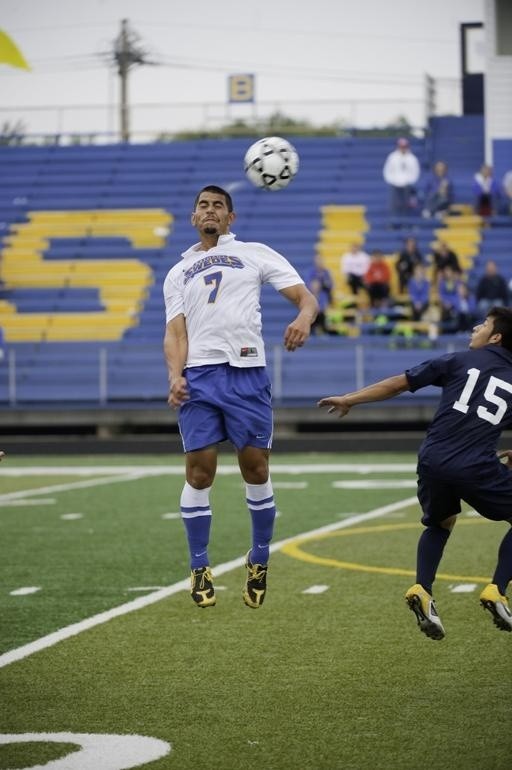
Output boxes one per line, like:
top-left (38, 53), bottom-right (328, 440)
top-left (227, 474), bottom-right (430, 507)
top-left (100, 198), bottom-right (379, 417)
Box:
top-left (0, 137), bottom-right (512, 406)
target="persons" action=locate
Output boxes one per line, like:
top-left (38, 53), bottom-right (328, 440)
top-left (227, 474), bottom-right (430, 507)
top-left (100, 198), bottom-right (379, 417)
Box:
top-left (164, 185), bottom-right (320, 610)
top-left (474, 164), bottom-right (511, 216)
top-left (310, 239), bottom-right (507, 341)
top-left (383, 139), bottom-right (452, 219)
top-left (317, 310), bottom-right (512, 639)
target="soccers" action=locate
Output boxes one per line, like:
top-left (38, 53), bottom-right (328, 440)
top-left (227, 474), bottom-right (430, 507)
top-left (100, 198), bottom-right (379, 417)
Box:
top-left (242, 135), bottom-right (298, 190)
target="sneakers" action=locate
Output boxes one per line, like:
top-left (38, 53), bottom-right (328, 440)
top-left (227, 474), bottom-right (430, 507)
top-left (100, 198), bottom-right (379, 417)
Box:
top-left (479, 584), bottom-right (512, 631)
top-left (241, 549), bottom-right (269, 609)
top-left (188, 565), bottom-right (216, 608)
top-left (405, 583), bottom-right (447, 640)
